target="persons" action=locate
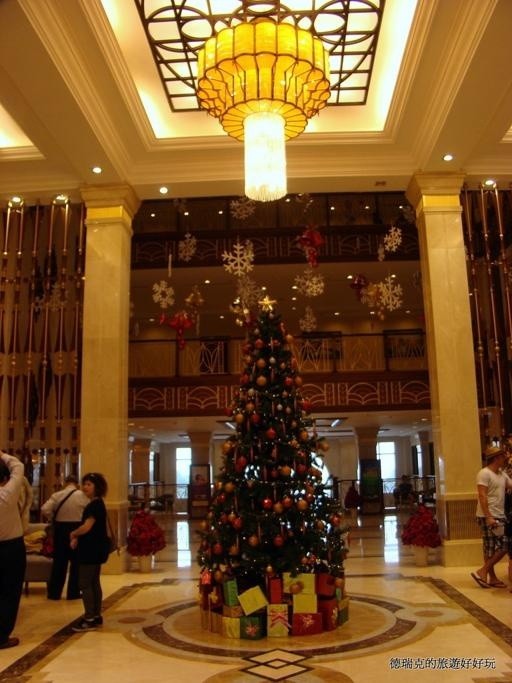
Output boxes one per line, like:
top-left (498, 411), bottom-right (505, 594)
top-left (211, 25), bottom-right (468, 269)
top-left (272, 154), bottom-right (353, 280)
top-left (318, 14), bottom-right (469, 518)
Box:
top-left (41, 474), bottom-right (91, 600)
top-left (71, 473), bottom-right (108, 632)
top-left (0, 451), bottom-right (26, 649)
top-left (471, 447), bottom-right (512, 588)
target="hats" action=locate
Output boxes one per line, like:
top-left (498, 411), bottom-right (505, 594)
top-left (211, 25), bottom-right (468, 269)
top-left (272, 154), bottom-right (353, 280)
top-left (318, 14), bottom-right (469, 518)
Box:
top-left (484, 446), bottom-right (505, 461)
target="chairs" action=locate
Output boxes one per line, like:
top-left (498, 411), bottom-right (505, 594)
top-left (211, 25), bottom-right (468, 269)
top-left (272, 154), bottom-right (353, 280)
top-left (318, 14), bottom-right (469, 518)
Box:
top-left (23, 522), bottom-right (53, 593)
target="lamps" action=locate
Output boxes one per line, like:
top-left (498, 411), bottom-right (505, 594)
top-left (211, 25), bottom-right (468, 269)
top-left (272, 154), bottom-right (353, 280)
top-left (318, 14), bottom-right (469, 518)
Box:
top-left (193, 13), bottom-right (332, 203)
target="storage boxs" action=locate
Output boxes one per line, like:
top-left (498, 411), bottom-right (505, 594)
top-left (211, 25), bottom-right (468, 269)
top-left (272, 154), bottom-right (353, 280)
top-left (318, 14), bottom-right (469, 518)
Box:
top-left (197, 570), bottom-right (349, 639)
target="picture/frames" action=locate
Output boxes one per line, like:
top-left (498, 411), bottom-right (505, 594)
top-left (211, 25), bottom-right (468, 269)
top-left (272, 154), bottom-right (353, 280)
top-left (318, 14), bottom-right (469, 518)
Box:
top-left (190, 463), bottom-right (210, 485)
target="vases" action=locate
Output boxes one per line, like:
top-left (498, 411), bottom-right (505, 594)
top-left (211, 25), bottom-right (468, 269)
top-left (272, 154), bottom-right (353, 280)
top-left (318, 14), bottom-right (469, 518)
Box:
top-left (138, 553), bottom-right (154, 572)
top-left (350, 508), bottom-right (358, 527)
top-left (411, 544), bottom-right (431, 567)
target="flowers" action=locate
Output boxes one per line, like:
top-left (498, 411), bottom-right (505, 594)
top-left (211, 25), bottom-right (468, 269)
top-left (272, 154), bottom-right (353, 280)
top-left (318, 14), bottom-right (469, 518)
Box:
top-left (343, 486), bottom-right (363, 508)
top-left (400, 502), bottom-right (444, 549)
top-left (125, 510), bottom-right (166, 557)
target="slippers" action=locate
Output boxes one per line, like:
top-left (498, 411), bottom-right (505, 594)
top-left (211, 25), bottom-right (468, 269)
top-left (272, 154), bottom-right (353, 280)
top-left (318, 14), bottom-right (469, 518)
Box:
top-left (490, 581), bottom-right (507, 587)
top-left (470, 572), bottom-right (489, 588)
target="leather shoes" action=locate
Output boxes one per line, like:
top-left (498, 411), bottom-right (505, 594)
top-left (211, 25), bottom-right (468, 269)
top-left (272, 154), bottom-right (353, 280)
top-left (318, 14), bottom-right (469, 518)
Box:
top-left (0, 637), bottom-right (19, 649)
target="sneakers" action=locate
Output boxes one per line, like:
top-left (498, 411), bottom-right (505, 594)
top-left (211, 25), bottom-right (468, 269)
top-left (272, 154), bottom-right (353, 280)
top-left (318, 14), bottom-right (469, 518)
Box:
top-left (71, 618), bottom-right (97, 632)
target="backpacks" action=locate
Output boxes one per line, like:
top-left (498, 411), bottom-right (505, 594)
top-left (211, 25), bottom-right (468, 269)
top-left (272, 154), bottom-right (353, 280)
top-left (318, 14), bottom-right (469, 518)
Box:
top-left (39, 526), bottom-right (54, 556)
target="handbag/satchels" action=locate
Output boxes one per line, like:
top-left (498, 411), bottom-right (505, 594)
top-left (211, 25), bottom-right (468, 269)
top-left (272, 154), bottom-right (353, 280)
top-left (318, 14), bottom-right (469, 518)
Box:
top-left (107, 535), bottom-right (120, 553)
